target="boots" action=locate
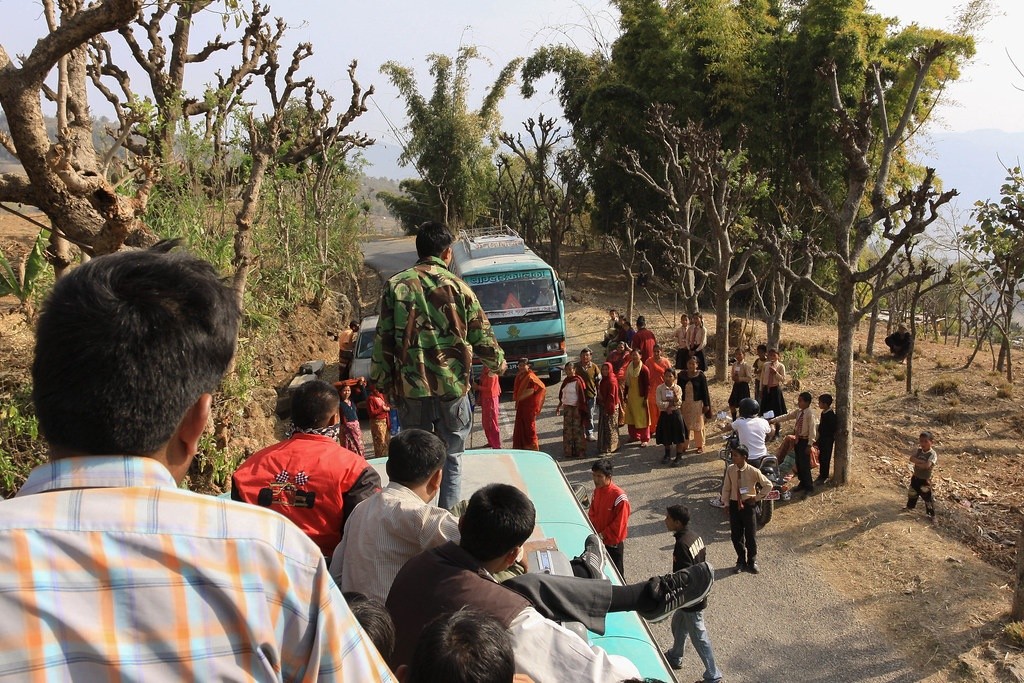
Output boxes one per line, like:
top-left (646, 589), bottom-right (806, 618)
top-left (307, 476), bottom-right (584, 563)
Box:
top-left (771, 425), bottom-right (780, 442)
top-left (765, 434), bottom-right (770, 443)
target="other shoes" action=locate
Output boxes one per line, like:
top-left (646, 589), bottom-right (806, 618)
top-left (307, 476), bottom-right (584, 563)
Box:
top-left (792, 485), bottom-right (804, 492)
top-left (734, 563), bottom-right (747, 573)
top-left (928, 515), bottom-right (938, 525)
top-left (482, 444), bottom-right (492, 448)
top-left (814, 476), bottom-right (830, 485)
top-left (564, 421), bottom-right (705, 467)
top-left (709, 499), bottom-right (721, 508)
top-left (902, 506), bottom-right (916, 513)
top-left (781, 490), bottom-right (792, 501)
top-left (800, 491), bottom-right (814, 500)
top-left (664, 650), bottom-right (682, 669)
top-left (695, 676), bottom-right (723, 683)
top-left (747, 562), bottom-right (759, 573)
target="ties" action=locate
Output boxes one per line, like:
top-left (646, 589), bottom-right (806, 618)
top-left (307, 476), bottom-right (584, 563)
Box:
top-left (691, 326), bottom-right (699, 349)
top-left (794, 411), bottom-right (803, 444)
top-left (737, 470), bottom-right (742, 512)
top-left (767, 364), bottom-right (774, 393)
top-left (734, 361), bottom-right (740, 377)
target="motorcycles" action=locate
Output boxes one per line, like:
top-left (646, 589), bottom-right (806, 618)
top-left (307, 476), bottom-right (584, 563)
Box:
top-left (714, 409), bottom-right (783, 525)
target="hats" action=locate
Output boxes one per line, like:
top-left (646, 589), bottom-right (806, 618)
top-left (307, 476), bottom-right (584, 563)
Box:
top-left (539, 280), bottom-right (548, 288)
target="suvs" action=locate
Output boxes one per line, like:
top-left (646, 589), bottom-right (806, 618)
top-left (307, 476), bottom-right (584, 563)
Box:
top-left (342, 315), bottom-right (387, 413)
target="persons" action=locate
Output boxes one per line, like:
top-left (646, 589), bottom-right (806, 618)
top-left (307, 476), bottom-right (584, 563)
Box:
top-left (884, 323), bottom-right (911, 360)
top-left (1, 221), bottom-right (790, 683)
top-left (813, 394), bottom-right (836, 484)
top-left (903, 431), bottom-right (939, 526)
top-left (768, 392), bottom-right (816, 494)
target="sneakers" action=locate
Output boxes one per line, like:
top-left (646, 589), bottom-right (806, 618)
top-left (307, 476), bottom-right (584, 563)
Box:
top-left (636, 561), bottom-right (714, 623)
top-left (570, 534), bottom-right (610, 581)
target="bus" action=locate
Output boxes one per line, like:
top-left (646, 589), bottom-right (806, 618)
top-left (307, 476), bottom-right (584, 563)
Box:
top-left (447, 224), bottom-right (569, 393)
top-left (214, 448), bottom-right (681, 683)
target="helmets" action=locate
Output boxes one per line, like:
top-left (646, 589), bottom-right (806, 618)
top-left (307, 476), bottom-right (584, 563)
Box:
top-left (739, 398), bottom-right (760, 418)
